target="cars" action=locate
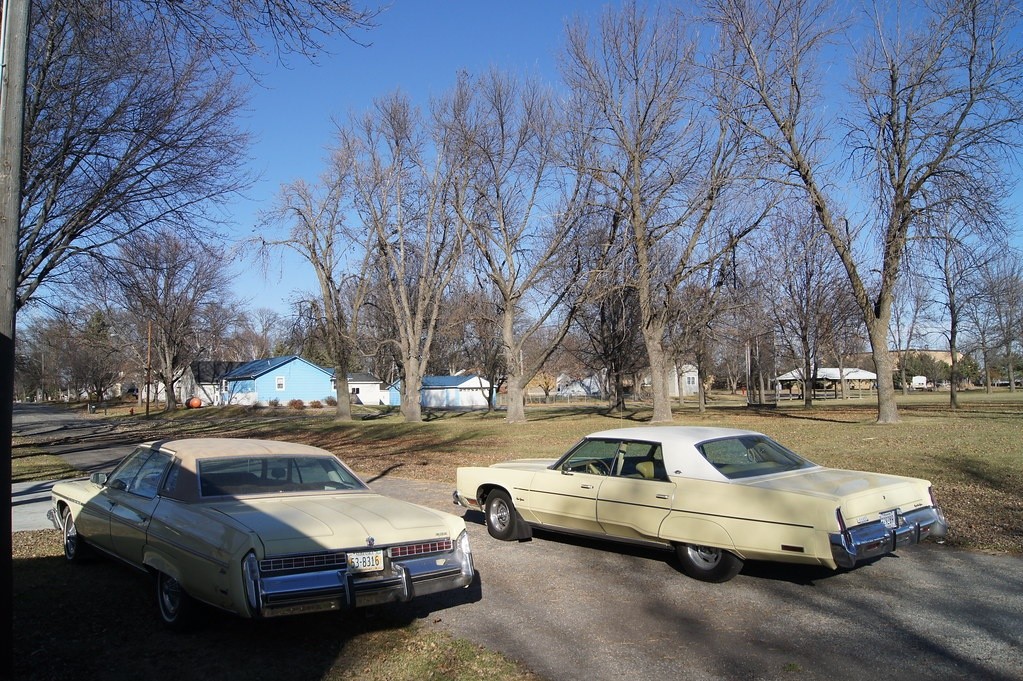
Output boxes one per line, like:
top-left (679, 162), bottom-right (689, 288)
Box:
top-left (455, 424), bottom-right (947, 592)
top-left (43, 435), bottom-right (477, 635)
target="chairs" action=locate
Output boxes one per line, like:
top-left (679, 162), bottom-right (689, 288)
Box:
top-left (632, 458), bottom-right (668, 482)
top-left (202, 478), bottom-right (318, 496)
